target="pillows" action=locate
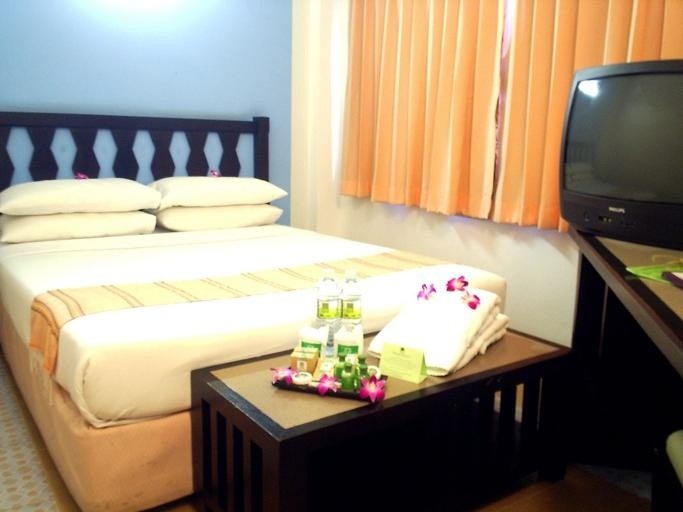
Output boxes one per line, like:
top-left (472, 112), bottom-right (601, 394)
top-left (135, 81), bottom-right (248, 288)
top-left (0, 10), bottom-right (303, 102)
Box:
top-left (0, 176), bottom-right (288, 245)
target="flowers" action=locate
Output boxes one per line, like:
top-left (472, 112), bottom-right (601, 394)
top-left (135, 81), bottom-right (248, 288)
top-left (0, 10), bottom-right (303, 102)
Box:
top-left (415, 275), bottom-right (482, 310)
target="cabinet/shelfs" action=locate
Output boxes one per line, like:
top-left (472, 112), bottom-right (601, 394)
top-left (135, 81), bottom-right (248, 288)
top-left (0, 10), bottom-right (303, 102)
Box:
top-left (189, 329), bottom-right (574, 512)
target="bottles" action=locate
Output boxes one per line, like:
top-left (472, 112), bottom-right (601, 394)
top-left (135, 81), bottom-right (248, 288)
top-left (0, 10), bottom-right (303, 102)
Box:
top-left (340, 270), bottom-right (362, 335)
top-left (316, 268), bottom-right (342, 357)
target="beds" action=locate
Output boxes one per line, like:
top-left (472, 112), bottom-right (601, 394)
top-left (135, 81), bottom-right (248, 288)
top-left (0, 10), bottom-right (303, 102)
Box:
top-left (0, 109), bottom-right (508, 512)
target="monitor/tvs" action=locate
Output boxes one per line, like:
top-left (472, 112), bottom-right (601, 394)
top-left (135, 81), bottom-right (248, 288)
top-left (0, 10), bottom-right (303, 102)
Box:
top-left (559, 59), bottom-right (683, 251)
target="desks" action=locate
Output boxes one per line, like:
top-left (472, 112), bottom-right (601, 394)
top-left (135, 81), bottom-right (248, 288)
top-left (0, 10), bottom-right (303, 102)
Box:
top-left (566, 220), bottom-right (683, 511)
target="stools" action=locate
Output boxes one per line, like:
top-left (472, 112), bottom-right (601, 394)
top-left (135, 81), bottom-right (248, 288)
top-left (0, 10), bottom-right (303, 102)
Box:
top-left (649, 430), bottom-right (683, 510)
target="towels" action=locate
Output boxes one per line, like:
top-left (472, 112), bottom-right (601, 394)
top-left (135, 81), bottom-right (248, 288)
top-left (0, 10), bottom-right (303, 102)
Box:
top-left (366, 285), bottom-right (508, 377)
